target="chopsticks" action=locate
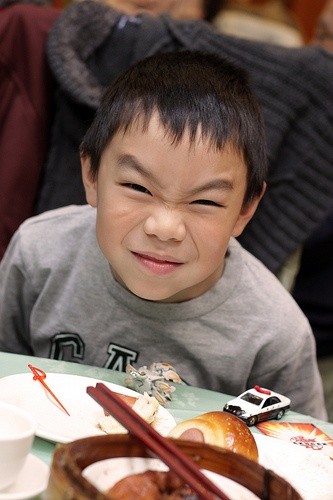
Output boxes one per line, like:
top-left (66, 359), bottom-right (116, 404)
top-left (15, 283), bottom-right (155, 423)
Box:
top-left (85, 383), bottom-right (229, 500)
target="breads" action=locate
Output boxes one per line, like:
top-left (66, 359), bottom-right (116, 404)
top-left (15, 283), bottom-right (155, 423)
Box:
top-left (166, 411), bottom-right (259, 464)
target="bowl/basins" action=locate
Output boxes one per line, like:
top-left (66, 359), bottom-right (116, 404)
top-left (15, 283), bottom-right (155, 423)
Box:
top-left (50, 434), bottom-right (302, 500)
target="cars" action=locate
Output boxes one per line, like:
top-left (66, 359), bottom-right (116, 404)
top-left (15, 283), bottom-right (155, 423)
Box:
top-left (223, 385), bottom-right (291, 427)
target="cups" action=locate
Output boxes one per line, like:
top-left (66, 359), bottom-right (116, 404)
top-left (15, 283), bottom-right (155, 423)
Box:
top-left (0, 409), bottom-right (36, 490)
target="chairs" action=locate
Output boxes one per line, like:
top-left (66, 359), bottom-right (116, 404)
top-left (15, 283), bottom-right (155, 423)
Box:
top-left (34, 1), bottom-right (333, 290)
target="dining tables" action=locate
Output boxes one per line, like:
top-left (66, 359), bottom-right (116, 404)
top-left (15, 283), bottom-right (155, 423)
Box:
top-left (1, 351), bottom-right (333, 500)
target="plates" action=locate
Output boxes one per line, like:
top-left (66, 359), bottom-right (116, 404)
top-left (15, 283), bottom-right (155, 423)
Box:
top-left (250, 434), bottom-right (333, 500)
top-left (0, 372), bottom-right (177, 445)
top-left (0, 454), bottom-right (50, 500)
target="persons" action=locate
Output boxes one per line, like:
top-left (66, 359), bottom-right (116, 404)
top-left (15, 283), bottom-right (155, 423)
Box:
top-left (0, 48), bottom-right (329, 422)
